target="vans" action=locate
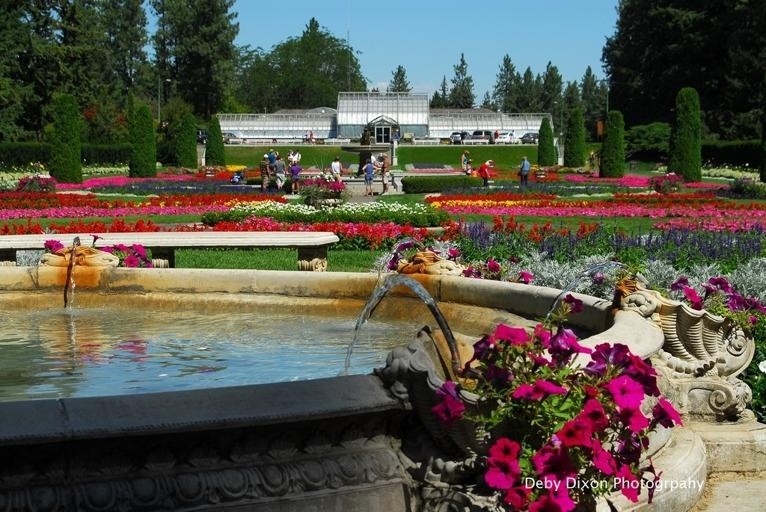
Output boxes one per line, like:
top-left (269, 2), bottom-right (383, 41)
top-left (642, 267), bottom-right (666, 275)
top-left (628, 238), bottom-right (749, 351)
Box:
top-left (472, 130), bottom-right (495, 144)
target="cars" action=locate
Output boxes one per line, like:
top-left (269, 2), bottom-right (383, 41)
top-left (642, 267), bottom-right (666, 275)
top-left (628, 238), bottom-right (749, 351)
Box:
top-left (450, 132), bottom-right (472, 145)
top-left (521, 132), bottom-right (539, 144)
top-left (222, 133), bottom-right (241, 144)
top-left (494, 131), bottom-right (521, 144)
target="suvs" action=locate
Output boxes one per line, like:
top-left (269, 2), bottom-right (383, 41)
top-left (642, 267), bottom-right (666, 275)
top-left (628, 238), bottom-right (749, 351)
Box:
top-left (196, 129), bottom-right (208, 144)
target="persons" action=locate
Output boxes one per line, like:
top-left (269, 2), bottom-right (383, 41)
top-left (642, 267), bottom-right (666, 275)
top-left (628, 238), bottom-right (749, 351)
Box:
top-left (494, 130), bottom-right (499, 145)
top-left (518, 157), bottom-right (530, 186)
top-left (461, 130), bottom-right (465, 145)
top-left (466, 159), bottom-right (473, 177)
top-left (260, 148), bottom-right (304, 196)
top-left (304, 130), bottom-right (309, 144)
top-left (308, 130), bottom-right (314, 144)
top-left (509, 130), bottom-right (515, 144)
top-left (362, 152), bottom-right (390, 197)
top-left (462, 150), bottom-right (470, 172)
top-left (331, 156), bottom-right (345, 193)
top-left (478, 161), bottom-right (491, 188)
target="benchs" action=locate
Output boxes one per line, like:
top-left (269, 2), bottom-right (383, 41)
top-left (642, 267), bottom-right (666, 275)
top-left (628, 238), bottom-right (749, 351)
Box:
top-left (0, 231), bottom-right (341, 273)
